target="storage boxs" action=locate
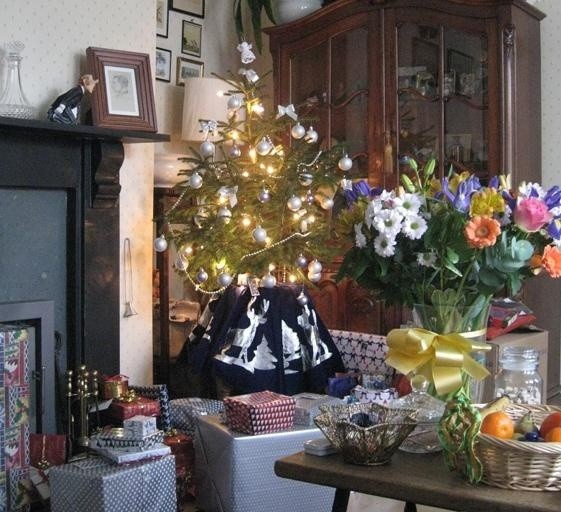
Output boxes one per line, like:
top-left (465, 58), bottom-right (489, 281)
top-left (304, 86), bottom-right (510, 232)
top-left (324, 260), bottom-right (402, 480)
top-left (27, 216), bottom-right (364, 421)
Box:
top-left (22, 387), bottom-right (359, 511)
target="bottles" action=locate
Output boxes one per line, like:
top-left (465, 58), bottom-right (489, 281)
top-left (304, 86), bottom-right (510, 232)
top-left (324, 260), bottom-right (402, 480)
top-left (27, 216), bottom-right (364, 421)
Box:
top-left (449, 136), bottom-right (464, 163)
top-left (493, 344), bottom-right (544, 405)
top-left (475, 140), bottom-right (490, 177)
top-left (0, 41), bottom-right (39, 120)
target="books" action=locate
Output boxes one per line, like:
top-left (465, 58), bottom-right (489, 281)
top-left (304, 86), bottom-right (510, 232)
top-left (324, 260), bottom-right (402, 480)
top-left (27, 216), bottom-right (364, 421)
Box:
top-left (98, 443), bottom-right (171, 467)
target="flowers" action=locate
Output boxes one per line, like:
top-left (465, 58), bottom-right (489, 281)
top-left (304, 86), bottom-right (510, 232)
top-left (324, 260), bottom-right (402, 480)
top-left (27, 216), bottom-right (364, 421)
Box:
top-left (343, 159), bottom-right (561, 288)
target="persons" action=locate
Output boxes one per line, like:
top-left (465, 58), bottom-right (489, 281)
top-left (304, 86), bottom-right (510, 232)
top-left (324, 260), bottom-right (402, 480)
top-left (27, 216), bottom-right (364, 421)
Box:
top-left (45, 73), bottom-right (99, 125)
top-left (109, 73), bottom-right (135, 112)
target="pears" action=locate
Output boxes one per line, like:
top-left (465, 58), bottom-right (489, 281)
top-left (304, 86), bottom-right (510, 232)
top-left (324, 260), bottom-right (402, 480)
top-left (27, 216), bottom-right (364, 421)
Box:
top-left (514, 410), bottom-right (540, 440)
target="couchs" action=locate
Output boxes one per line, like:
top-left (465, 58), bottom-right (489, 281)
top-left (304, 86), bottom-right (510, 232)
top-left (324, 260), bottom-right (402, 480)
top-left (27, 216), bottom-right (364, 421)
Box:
top-left (165, 329), bottom-right (414, 434)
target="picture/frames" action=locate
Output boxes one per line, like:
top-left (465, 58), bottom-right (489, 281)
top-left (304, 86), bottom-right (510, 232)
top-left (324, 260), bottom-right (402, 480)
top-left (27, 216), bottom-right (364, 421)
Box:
top-left (156, 1), bottom-right (208, 87)
top-left (83, 46), bottom-right (159, 134)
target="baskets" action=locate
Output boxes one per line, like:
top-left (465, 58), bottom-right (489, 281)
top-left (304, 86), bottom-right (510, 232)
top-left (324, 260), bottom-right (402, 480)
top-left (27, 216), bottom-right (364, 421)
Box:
top-left (313, 399), bottom-right (420, 467)
top-left (439, 403), bottom-right (561, 492)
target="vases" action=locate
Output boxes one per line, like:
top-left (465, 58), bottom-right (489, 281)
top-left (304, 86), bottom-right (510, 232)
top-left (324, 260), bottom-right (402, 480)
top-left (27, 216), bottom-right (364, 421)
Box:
top-left (151, 159), bottom-right (184, 192)
top-left (409, 298), bottom-right (496, 405)
top-left (268, 0), bottom-right (324, 26)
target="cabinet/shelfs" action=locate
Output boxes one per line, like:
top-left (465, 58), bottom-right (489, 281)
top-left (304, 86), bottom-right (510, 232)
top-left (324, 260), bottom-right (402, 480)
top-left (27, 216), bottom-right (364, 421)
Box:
top-left (254, 0), bottom-right (550, 335)
top-left (154, 188), bottom-right (211, 381)
top-left (1, 110), bottom-right (125, 511)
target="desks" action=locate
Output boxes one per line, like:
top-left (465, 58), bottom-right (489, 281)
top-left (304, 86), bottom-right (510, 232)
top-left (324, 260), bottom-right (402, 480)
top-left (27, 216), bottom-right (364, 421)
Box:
top-left (274, 419), bottom-right (561, 512)
top-left (468, 318), bottom-right (552, 407)
top-left (184, 277), bottom-right (347, 401)
